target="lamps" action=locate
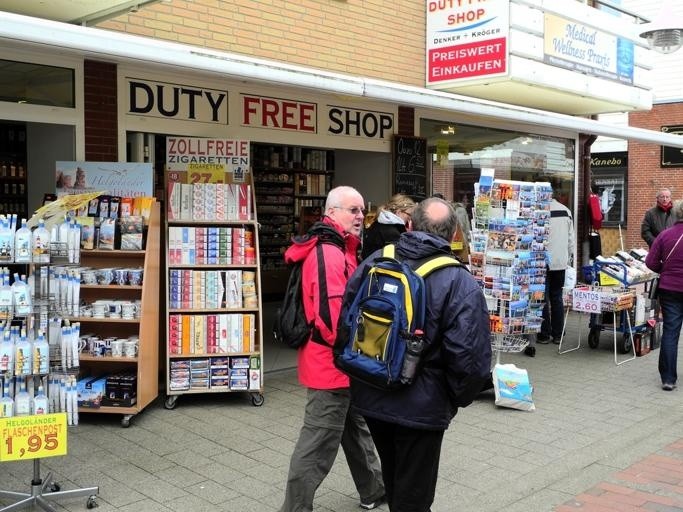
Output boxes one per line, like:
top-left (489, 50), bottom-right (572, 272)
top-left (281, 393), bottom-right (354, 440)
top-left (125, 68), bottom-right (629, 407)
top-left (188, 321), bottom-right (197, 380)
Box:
top-left (593, 0), bottom-right (682, 53)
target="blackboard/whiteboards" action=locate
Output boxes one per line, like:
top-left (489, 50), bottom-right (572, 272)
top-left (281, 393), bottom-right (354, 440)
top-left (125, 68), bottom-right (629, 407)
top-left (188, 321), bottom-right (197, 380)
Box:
top-left (392, 134), bottom-right (430, 203)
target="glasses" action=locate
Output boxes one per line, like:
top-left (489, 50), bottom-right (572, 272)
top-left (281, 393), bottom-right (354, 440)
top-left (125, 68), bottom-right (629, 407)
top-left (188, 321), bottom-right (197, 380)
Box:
top-left (332, 206), bottom-right (368, 215)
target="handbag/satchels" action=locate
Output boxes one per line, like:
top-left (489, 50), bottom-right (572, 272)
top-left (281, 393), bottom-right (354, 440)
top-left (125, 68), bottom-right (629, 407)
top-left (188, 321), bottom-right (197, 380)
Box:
top-left (492, 363), bottom-right (536, 413)
top-left (277, 264), bottom-right (315, 349)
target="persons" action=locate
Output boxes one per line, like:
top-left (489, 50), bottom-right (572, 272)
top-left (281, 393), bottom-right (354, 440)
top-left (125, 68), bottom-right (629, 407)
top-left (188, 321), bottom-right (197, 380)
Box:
top-left (514, 324), bottom-right (537, 358)
top-left (534, 189), bottom-right (576, 344)
top-left (55, 169), bottom-right (64, 189)
top-left (82, 224), bottom-right (91, 242)
top-left (347, 196), bottom-right (491, 511)
top-left (73, 166), bottom-right (85, 190)
top-left (641, 187), bottom-right (675, 252)
top-left (355, 191), bottom-right (476, 266)
top-left (644, 199), bottom-right (682, 390)
top-left (277, 185), bottom-right (386, 511)
top-left (63, 174), bottom-right (72, 189)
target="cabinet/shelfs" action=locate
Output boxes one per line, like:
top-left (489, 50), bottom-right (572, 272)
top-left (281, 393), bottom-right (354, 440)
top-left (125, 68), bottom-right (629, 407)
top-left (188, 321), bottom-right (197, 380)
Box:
top-left (467, 180), bottom-right (553, 374)
top-left (33, 201), bottom-right (160, 428)
top-left (250, 144), bottom-right (334, 294)
top-left (0, 224), bottom-right (99, 511)
top-left (162, 164), bottom-right (264, 409)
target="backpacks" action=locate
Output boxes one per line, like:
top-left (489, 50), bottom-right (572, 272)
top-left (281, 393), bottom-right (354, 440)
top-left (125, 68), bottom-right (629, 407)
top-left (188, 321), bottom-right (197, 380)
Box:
top-left (334, 244), bottom-right (472, 398)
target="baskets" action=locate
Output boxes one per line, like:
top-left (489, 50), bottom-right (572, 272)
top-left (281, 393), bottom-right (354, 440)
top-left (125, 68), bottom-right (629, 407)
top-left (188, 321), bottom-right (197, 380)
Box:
top-left (562, 281), bottom-right (637, 313)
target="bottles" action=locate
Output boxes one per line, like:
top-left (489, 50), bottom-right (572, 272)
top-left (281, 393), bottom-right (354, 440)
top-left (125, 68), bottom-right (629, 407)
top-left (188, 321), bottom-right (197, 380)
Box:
top-left (399, 328), bottom-right (426, 386)
top-left (0, 212), bottom-right (52, 417)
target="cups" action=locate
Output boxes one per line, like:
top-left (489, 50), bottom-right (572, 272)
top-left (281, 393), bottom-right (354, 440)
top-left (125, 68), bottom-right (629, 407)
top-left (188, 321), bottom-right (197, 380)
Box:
top-left (82, 267), bottom-right (144, 358)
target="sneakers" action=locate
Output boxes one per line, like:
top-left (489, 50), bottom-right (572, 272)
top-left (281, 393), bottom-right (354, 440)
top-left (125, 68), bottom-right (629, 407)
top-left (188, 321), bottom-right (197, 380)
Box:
top-left (662, 382), bottom-right (677, 390)
top-left (537, 338), bottom-right (561, 344)
top-left (359, 498), bottom-right (383, 509)
